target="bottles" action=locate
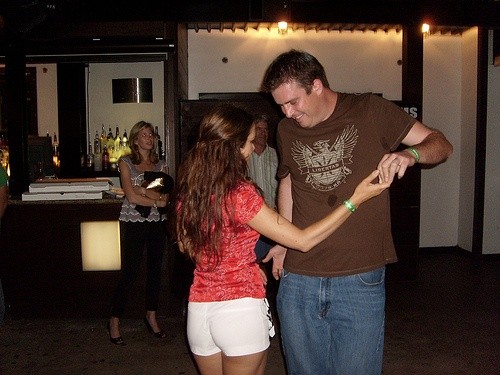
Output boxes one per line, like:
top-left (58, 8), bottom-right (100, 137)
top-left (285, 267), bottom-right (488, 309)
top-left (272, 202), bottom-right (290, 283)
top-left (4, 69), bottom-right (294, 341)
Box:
top-left (121, 128), bottom-right (128, 147)
top-left (114, 124), bottom-right (121, 142)
top-left (93, 130), bottom-right (100, 154)
top-left (154, 126), bottom-right (161, 145)
top-left (100, 124), bottom-right (107, 142)
top-left (86, 144), bottom-right (95, 172)
top-left (106, 128), bottom-right (115, 142)
top-left (53, 132), bottom-right (60, 157)
top-left (102, 147), bottom-right (109, 171)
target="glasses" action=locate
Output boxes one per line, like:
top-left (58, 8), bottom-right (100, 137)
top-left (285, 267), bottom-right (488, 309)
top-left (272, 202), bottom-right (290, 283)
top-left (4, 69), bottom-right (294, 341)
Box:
top-left (138, 133), bottom-right (154, 139)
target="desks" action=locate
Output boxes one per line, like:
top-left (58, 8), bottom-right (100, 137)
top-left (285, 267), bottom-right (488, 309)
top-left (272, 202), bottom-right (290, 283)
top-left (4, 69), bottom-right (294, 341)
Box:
top-left (0, 196), bottom-right (124, 316)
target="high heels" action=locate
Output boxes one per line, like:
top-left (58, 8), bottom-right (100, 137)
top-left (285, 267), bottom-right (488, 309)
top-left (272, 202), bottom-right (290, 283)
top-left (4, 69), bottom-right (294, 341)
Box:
top-left (106, 320), bottom-right (123, 345)
top-left (144, 316), bottom-right (166, 338)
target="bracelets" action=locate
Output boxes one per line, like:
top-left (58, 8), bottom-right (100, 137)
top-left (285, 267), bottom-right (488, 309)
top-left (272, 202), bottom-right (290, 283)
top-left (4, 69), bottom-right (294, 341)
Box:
top-left (158, 194), bottom-right (162, 201)
top-left (409, 147), bottom-right (421, 163)
top-left (342, 200), bottom-right (357, 213)
top-left (153, 200), bottom-right (157, 208)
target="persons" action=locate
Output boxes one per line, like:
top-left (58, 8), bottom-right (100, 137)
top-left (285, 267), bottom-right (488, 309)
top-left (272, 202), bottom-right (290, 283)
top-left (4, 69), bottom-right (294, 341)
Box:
top-left (0, 163), bottom-right (9, 219)
top-left (174, 109), bottom-right (392, 375)
top-left (260, 49), bottom-right (453, 375)
top-left (244, 114), bottom-right (278, 312)
top-left (106, 122), bottom-right (169, 345)
